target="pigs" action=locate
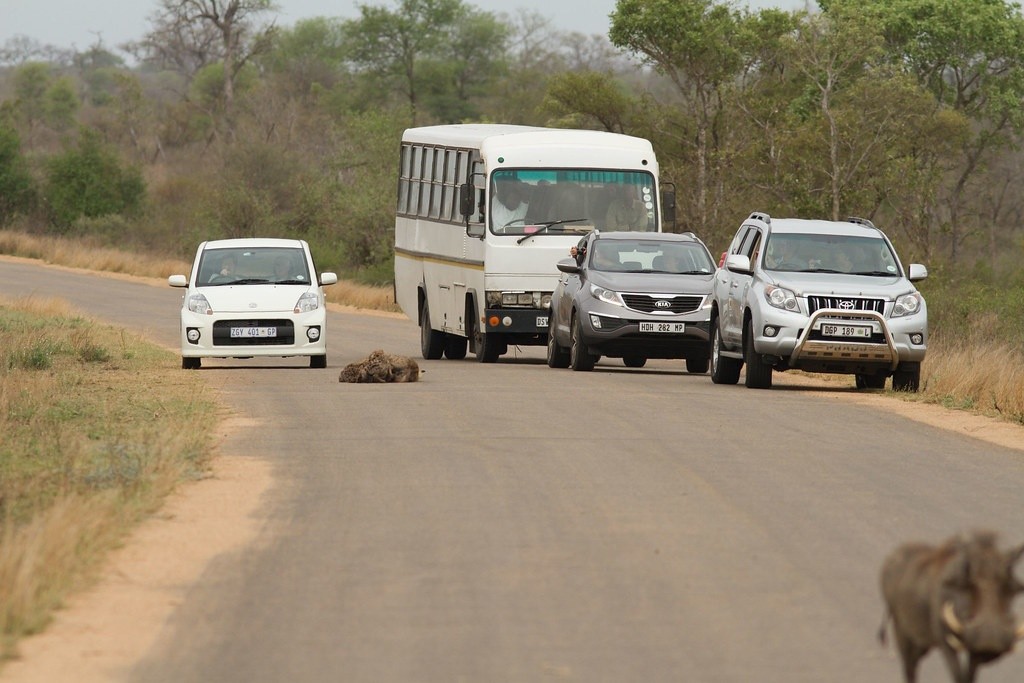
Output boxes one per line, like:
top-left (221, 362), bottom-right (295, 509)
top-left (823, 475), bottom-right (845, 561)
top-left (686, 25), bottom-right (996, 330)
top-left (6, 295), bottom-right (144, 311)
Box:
top-left (876, 530), bottom-right (1024, 683)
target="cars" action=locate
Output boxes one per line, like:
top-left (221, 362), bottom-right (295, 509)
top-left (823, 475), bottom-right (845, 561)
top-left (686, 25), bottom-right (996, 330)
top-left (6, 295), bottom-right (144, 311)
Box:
top-left (545, 230), bottom-right (720, 373)
top-left (166, 237), bottom-right (338, 370)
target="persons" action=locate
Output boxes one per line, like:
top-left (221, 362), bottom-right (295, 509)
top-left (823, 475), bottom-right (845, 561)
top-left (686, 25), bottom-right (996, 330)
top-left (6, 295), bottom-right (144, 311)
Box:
top-left (573, 244), bottom-right (618, 270)
top-left (493, 174), bottom-right (648, 234)
top-left (659, 247), bottom-right (687, 274)
top-left (206, 253), bottom-right (300, 285)
top-left (768, 233), bottom-right (884, 276)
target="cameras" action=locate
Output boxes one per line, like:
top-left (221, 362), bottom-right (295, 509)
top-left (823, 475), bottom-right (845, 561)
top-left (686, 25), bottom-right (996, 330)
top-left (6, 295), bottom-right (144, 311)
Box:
top-left (577, 249), bottom-right (584, 256)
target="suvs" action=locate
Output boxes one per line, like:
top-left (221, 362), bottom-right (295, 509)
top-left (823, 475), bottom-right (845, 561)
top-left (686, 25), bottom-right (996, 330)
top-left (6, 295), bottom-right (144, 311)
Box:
top-left (707, 211), bottom-right (928, 395)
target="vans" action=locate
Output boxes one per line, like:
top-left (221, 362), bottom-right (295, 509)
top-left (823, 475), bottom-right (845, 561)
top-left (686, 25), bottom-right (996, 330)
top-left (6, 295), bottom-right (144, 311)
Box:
top-left (392, 122), bottom-right (678, 363)
